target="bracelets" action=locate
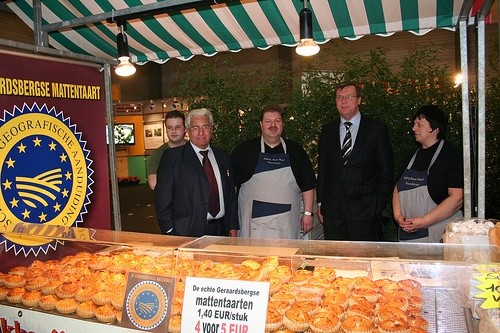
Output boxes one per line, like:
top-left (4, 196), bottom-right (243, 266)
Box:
top-left (301, 211), bottom-right (314, 216)
top-left (317, 203), bottom-right (321, 207)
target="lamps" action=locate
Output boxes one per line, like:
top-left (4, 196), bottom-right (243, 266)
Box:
top-left (115, 21), bottom-right (136, 76)
top-left (295, 0), bottom-right (320, 56)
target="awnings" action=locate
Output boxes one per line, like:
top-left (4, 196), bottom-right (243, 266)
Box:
top-left (0, 0), bottom-right (493, 65)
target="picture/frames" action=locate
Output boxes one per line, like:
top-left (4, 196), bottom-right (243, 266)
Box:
top-left (106, 123), bottom-right (136, 146)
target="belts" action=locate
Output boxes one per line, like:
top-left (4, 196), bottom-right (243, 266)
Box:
top-left (209, 220), bottom-right (218, 224)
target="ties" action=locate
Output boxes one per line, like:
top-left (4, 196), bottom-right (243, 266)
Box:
top-left (341, 122), bottom-right (353, 166)
top-left (199, 150), bottom-right (221, 218)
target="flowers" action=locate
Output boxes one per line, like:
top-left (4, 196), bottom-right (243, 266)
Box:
top-left (117, 175), bottom-right (140, 186)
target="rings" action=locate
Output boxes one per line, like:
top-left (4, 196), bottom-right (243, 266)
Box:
top-left (402, 227), bottom-right (405, 230)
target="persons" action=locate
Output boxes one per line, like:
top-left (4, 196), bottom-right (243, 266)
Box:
top-left (148, 110), bottom-right (189, 191)
top-left (393, 105), bottom-right (463, 243)
top-left (153, 107), bottom-right (240, 237)
top-left (230, 106), bottom-right (315, 239)
top-left (316, 83), bottom-right (395, 242)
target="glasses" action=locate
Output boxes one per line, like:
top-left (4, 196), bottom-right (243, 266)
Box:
top-left (188, 125), bottom-right (210, 132)
top-left (337, 94), bottom-right (358, 100)
